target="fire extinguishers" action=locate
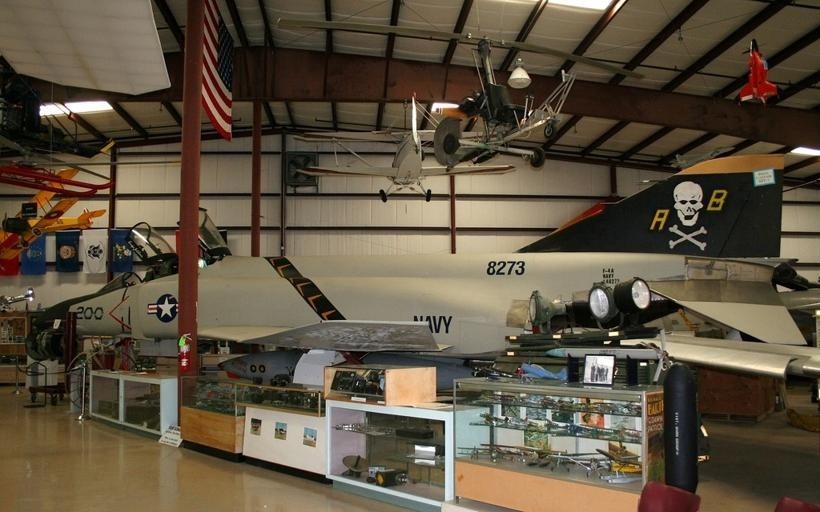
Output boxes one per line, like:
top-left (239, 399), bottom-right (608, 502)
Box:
top-left (178, 333), bottom-right (192, 373)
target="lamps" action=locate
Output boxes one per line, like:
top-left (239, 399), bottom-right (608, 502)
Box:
top-left (507, 56), bottom-right (533, 91)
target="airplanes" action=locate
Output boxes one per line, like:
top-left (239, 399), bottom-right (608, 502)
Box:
top-left (24, 153), bottom-right (820, 386)
top-left (736, 39), bottom-right (777, 106)
top-left (276, 13), bottom-right (646, 203)
top-left (0, 163), bottom-right (114, 261)
top-left (458, 444), bottom-right (643, 484)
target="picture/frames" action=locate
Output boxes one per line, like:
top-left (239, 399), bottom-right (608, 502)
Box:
top-left (583, 353), bottom-right (615, 387)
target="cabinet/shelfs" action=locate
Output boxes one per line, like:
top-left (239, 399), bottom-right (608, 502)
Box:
top-left (243, 379), bottom-right (326, 487)
top-left (181, 375), bottom-right (245, 479)
top-left (326, 398), bottom-right (489, 512)
top-left (323, 361), bottom-right (436, 405)
top-left (88, 371), bottom-right (179, 440)
top-left (453, 376), bottom-right (666, 512)
top-left (0, 307), bottom-right (37, 384)
